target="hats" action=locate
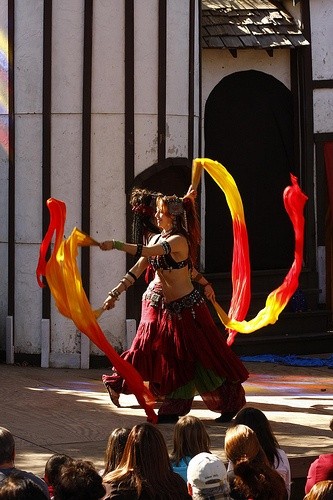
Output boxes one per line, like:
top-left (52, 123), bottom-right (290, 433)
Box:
top-left (187, 452), bottom-right (228, 489)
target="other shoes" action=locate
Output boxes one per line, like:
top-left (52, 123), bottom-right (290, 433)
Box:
top-left (101, 373), bottom-right (122, 408)
top-left (215, 399), bottom-right (246, 423)
top-left (147, 414), bottom-right (179, 424)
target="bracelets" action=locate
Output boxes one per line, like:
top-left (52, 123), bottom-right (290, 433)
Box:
top-left (112, 239), bottom-right (143, 255)
top-left (108, 271), bottom-right (138, 301)
top-left (193, 273), bottom-right (212, 287)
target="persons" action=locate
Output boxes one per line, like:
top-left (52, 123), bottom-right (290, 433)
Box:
top-left (99, 190), bottom-right (250, 421)
top-left (0, 406), bottom-right (333, 500)
